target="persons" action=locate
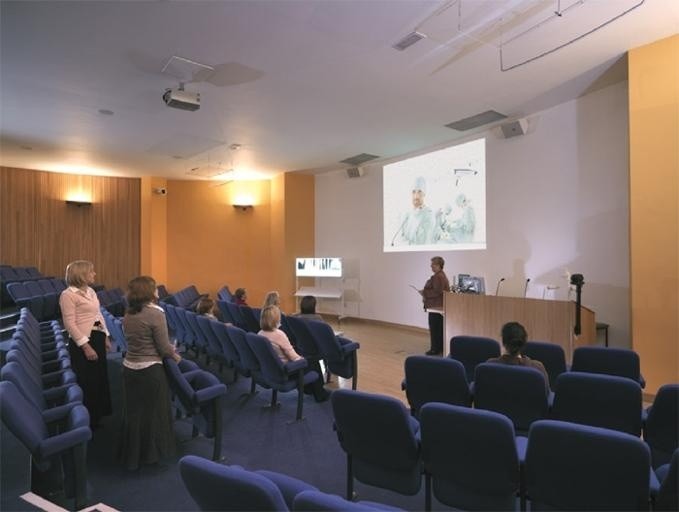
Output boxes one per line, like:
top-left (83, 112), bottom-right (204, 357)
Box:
top-left (256, 305), bottom-right (332, 403)
top-left (290, 295), bottom-right (353, 345)
top-left (58, 260), bottom-right (112, 462)
top-left (233, 286), bottom-right (249, 304)
top-left (417, 256), bottom-right (449, 356)
top-left (195, 296), bottom-right (219, 322)
top-left (262, 290), bottom-right (283, 309)
top-left (469, 321), bottom-right (552, 404)
top-left (116, 275), bottom-right (180, 474)
top-left (393, 176), bottom-right (475, 245)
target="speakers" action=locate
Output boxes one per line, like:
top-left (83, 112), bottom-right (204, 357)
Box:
top-left (500, 119), bottom-right (529, 138)
top-left (346, 167), bottom-right (363, 178)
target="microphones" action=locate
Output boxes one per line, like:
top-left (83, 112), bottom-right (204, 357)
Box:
top-left (524, 278), bottom-right (531, 298)
top-left (495, 277), bottom-right (505, 296)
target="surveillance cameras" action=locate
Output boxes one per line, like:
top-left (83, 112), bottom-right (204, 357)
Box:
top-left (159, 187), bottom-right (167, 196)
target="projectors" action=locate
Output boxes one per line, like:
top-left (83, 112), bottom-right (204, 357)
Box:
top-left (163, 86), bottom-right (201, 112)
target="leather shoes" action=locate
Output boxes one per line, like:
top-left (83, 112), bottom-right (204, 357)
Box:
top-left (426, 349), bottom-right (439, 355)
top-left (316, 389), bottom-right (332, 402)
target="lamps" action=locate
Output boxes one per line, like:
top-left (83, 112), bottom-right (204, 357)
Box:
top-left (233, 205), bottom-right (254, 212)
top-left (65, 201), bottom-right (92, 208)
